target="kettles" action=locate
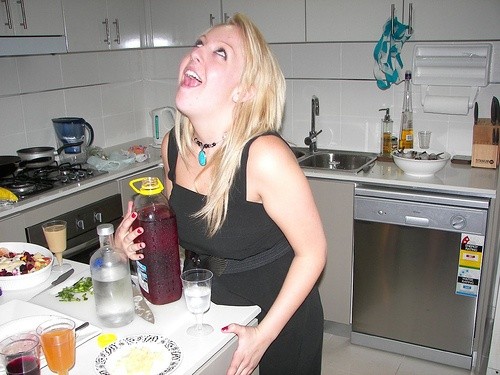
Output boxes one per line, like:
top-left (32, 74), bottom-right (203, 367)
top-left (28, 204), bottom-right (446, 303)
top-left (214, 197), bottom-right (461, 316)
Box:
top-left (149, 106), bottom-right (177, 149)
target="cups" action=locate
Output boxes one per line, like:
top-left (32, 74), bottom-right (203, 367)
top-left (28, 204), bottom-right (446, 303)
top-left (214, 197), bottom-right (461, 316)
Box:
top-left (418, 131), bottom-right (431, 149)
top-left (0, 333), bottom-right (42, 375)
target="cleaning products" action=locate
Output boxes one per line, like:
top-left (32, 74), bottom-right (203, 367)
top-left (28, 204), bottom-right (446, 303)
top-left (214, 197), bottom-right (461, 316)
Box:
top-left (378, 108), bottom-right (393, 160)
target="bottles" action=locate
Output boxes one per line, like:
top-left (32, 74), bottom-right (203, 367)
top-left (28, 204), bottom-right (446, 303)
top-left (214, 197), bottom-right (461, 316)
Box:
top-left (399, 70), bottom-right (414, 149)
top-left (89, 224), bottom-right (136, 328)
top-left (392, 137), bottom-right (398, 152)
top-left (132, 176), bottom-right (182, 305)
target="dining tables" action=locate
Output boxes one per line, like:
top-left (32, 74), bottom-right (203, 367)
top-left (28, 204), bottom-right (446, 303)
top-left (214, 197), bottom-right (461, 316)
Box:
top-left (0, 257), bottom-right (260, 375)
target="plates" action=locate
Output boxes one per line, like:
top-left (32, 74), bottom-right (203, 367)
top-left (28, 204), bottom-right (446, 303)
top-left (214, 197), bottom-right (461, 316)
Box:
top-left (93, 334), bottom-right (183, 375)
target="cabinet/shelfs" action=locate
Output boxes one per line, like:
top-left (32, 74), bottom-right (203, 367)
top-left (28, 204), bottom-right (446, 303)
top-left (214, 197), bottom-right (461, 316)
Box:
top-left (305, 0), bottom-right (500, 43)
top-left (0, 0), bottom-right (64, 36)
top-left (150, 0), bottom-right (306, 48)
top-left (62, 0), bottom-right (146, 53)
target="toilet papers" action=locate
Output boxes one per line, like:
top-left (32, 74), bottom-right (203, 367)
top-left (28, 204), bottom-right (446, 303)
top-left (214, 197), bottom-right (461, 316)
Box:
top-left (423, 95), bottom-right (469, 116)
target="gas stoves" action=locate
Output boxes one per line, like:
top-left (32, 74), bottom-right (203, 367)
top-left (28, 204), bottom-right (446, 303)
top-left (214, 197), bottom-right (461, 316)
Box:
top-left (0, 164), bottom-right (109, 206)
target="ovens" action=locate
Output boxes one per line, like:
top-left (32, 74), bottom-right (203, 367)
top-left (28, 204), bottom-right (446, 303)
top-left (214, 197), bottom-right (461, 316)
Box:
top-left (26, 194), bottom-right (124, 265)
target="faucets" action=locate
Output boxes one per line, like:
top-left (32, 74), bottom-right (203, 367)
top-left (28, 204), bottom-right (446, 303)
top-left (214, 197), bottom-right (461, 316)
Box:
top-left (303, 94), bottom-right (323, 152)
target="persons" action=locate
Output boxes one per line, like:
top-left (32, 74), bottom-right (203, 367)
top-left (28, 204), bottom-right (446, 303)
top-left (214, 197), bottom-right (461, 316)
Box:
top-left (113, 11), bottom-right (329, 375)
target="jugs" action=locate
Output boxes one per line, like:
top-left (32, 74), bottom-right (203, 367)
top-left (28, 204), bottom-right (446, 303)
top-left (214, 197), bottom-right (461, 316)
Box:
top-left (52, 117), bottom-right (94, 164)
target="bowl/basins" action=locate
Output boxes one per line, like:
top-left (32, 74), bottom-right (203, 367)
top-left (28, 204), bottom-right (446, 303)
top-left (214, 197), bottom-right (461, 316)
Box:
top-left (0, 242), bottom-right (54, 291)
top-left (391, 148), bottom-right (452, 177)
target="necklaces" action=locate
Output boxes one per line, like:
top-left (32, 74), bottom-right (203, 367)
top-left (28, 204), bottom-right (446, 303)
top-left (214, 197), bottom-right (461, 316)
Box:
top-left (192, 137), bottom-right (219, 167)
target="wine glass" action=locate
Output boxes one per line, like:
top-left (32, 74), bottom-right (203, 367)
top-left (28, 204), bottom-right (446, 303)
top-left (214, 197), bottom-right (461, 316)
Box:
top-left (36, 317), bottom-right (76, 375)
top-left (180, 268), bottom-right (214, 338)
top-left (41, 220), bottom-right (71, 271)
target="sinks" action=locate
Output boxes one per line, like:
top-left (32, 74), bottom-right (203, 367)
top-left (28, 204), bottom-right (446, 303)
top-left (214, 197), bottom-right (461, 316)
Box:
top-left (292, 147), bottom-right (305, 159)
top-left (298, 153), bottom-right (378, 174)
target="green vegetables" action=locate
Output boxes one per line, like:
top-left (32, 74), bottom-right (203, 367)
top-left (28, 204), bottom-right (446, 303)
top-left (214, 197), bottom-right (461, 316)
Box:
top-left (56, 277), bottom-right (94, 302)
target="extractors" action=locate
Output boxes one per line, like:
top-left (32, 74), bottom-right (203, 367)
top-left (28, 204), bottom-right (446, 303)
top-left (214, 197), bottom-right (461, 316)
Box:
top-left (0, 36), bottom-right (69, 58)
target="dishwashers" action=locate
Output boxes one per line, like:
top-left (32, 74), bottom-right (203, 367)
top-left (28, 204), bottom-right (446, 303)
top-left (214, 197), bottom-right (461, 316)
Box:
top-left (349, 185), bottom-right (491, 371)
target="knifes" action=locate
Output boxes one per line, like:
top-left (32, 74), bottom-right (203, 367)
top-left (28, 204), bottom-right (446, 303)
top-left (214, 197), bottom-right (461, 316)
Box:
top-left (491, 96), bottom-right (500, 146)
top-left (474, 102), bottom-right (479, 125)
top-left (131, 279), bottom-right (155, 325)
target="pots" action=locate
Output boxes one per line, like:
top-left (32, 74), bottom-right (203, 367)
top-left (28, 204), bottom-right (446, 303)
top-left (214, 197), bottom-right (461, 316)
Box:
top-left (0, 141), bottom-right (84, 178)
